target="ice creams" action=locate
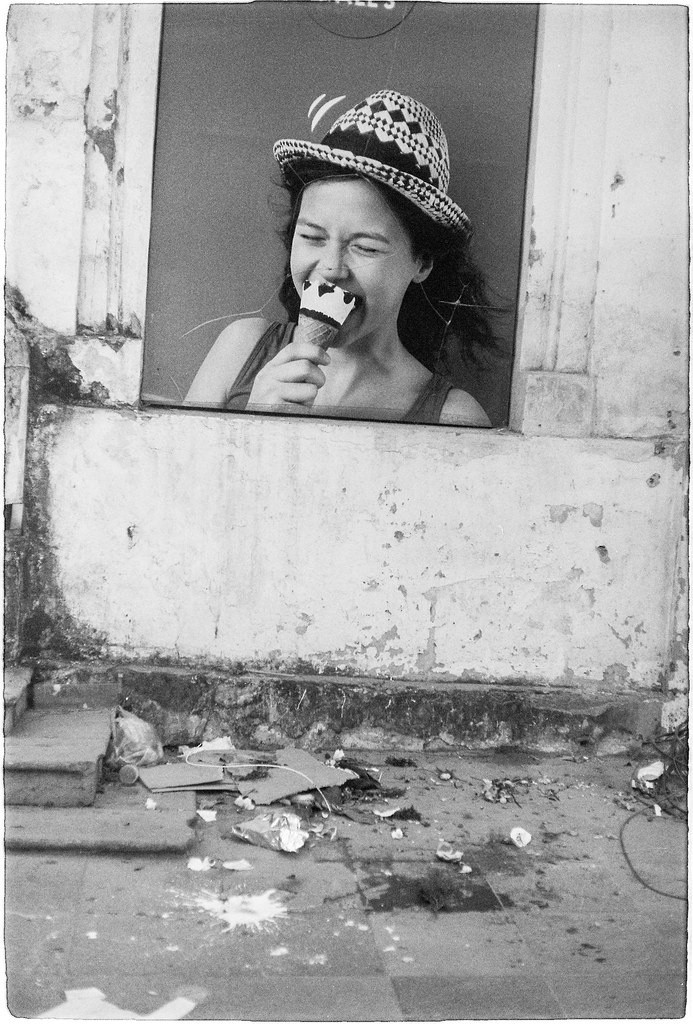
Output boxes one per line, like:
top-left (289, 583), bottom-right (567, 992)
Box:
top-left (297, 280), bottom-right (357, 354)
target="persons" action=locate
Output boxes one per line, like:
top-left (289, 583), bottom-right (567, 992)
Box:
top-left (181, 89), bottom-right (492, 426)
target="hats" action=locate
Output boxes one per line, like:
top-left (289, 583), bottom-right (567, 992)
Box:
top-left (273, 89), bottom-right (474, 241)
